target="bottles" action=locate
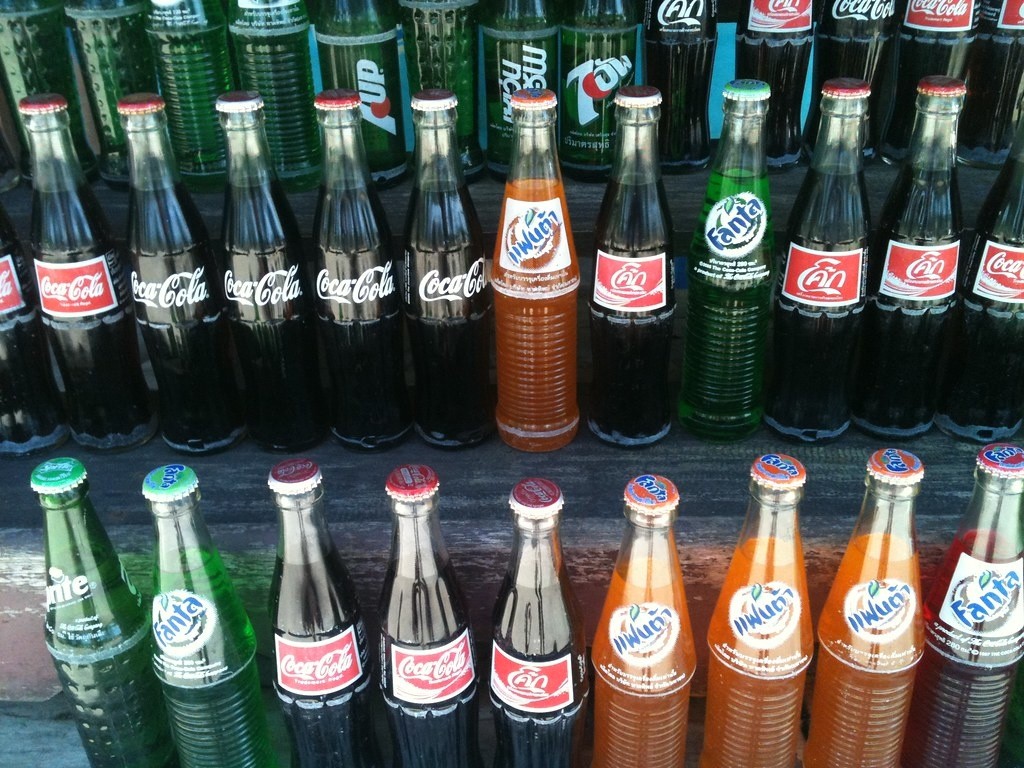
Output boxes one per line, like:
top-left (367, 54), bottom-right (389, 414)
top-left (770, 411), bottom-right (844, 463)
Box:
top-left (645, 0), bottom-right (811, 171)
top-left (396, 0), bottom-right (641, 184)
top-left (799, 0), bottom-right (1023, 169)
top-left (379, 463), bottom-right (482, 768)
top-left (585, 84), bottom-right (677, 449)
top-left (898, 443), bottom-right (1024, 768)
top-left (29, 457), bottom-right (179, 768)
top-left (1, 0), bottom-right (407, 193)
top-left (119, 93), bottom-right (247, 455)
top-left (143, 462), bottom-right (275, 768)
top-left (802, 448), bottom-right (926, 768)
top-left (492, 89), bottom-right (582, 452)
top-left (933, 112), bottom-right (1024, 447)
top-left (402, 92), bottom-right (497, 452)
top-left (266, 458), bottom-right (389, 768)
top-left (680, 79), bottom-right (773, 441)
top-left (1, 204), bottom-right (70, 458)
top-left (852, 76), bottom-right (969, 443)
top-left (15, 92), bottom-right (157, 450)
top-left (311, 90), bottom-right (416, 450)
top-left (696, 453), bottom-right (816, 768)
top-left (764, 79), bottom-right (871, 449)
top-left (489, 480), bottom-right (590, 768)
top-left (591, 475), bottom-right (699, 768)
top-left (209, 93), bottom-right (328, 454)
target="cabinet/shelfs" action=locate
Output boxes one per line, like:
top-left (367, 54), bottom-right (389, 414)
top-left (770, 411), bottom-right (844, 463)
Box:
top-left (0, 138), bottom-right (1024, 768)
